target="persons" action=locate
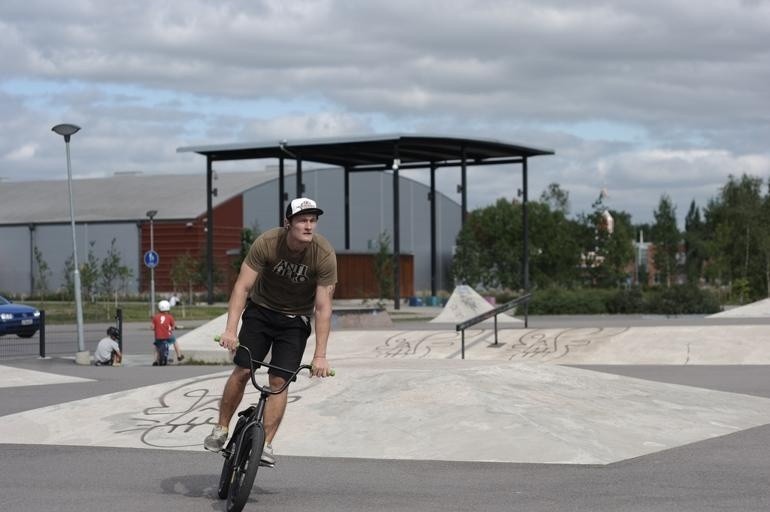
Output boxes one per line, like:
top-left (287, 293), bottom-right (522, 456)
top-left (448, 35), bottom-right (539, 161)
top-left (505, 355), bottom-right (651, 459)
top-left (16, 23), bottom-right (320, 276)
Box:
top-left (204, 198), bottom-right (339, 465)
top-left (94, 327), bottom-right (122, 367)
top-left (150, 299), bottom-right (184, 366)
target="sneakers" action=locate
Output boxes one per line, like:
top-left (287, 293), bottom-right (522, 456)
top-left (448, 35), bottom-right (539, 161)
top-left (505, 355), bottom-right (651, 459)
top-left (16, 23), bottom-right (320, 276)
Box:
top-left (260, 441), bottom-right (276, 463)
top-left (204, 428), bottom-right (228, 452)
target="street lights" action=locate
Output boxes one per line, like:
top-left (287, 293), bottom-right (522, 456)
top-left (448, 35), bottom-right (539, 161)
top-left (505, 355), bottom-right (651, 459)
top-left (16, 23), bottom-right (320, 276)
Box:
top-left (145, 208), bottom-right (160, 319)
top-left (51, 122), bottom-right (91, 366)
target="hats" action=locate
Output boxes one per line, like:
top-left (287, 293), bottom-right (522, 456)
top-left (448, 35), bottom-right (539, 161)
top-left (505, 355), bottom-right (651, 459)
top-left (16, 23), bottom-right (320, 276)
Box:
top-left (286, 198), bottom-right (323, 216)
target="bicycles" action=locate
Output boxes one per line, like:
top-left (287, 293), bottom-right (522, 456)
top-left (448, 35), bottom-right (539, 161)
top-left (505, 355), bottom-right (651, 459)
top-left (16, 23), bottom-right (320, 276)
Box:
top-left (158, 340), bottom-right (172, 365)
top-left (210, 334), bottom-right (336, 512)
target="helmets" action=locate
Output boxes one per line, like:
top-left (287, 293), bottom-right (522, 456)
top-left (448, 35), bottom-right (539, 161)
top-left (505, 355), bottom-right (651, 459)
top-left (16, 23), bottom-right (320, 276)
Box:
top-left (158, 300), bottom-right (170, 312)
top-left (108, 327), bottom-right (120, 339)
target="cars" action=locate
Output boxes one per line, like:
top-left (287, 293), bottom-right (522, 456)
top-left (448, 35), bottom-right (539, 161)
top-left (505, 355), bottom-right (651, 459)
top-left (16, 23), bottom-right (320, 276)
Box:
top-left (0, 294), bottom-right (41, 338)
